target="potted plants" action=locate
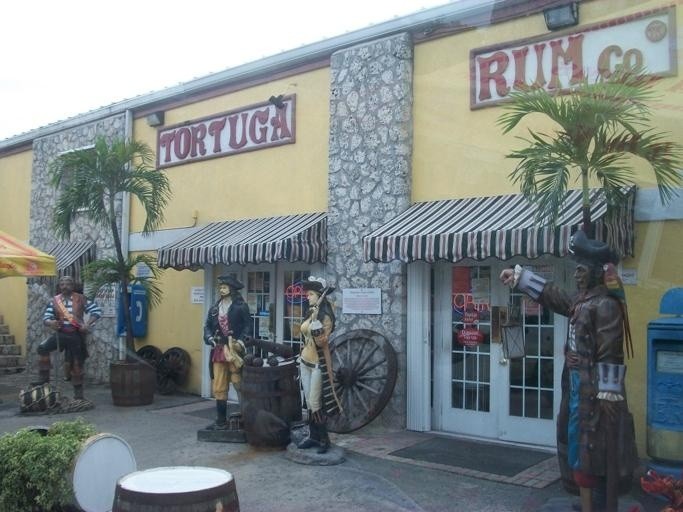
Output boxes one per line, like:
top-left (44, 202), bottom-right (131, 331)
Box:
top-left (46, 132), bottom-right (173, 407)
top-left (495, 61), bottom-right (683, 500)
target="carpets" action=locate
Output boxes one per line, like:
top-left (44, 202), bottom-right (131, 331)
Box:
top-left (146, 400), bottom-right (307, 431)
top-left (345, 431), bottom-right (564, 491)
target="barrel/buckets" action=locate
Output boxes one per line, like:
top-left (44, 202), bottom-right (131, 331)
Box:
top-left (111, 465), bottom-right (240, 512)
top-left (13, 426), bottom-right (136, 512)
top-left (240, 358), bottom-right (301, 449)
top-left (110, 360), bottom-right (154, 406)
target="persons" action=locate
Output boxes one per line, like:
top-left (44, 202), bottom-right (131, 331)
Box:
top-left (297, 279), bottom-right (335, 454)
top-left (498, 260), bottom-right (628, 512)
top-left (29, 275), bottom-right (104, 400)
top-left (204, 273), bottom-right (251, 431)
